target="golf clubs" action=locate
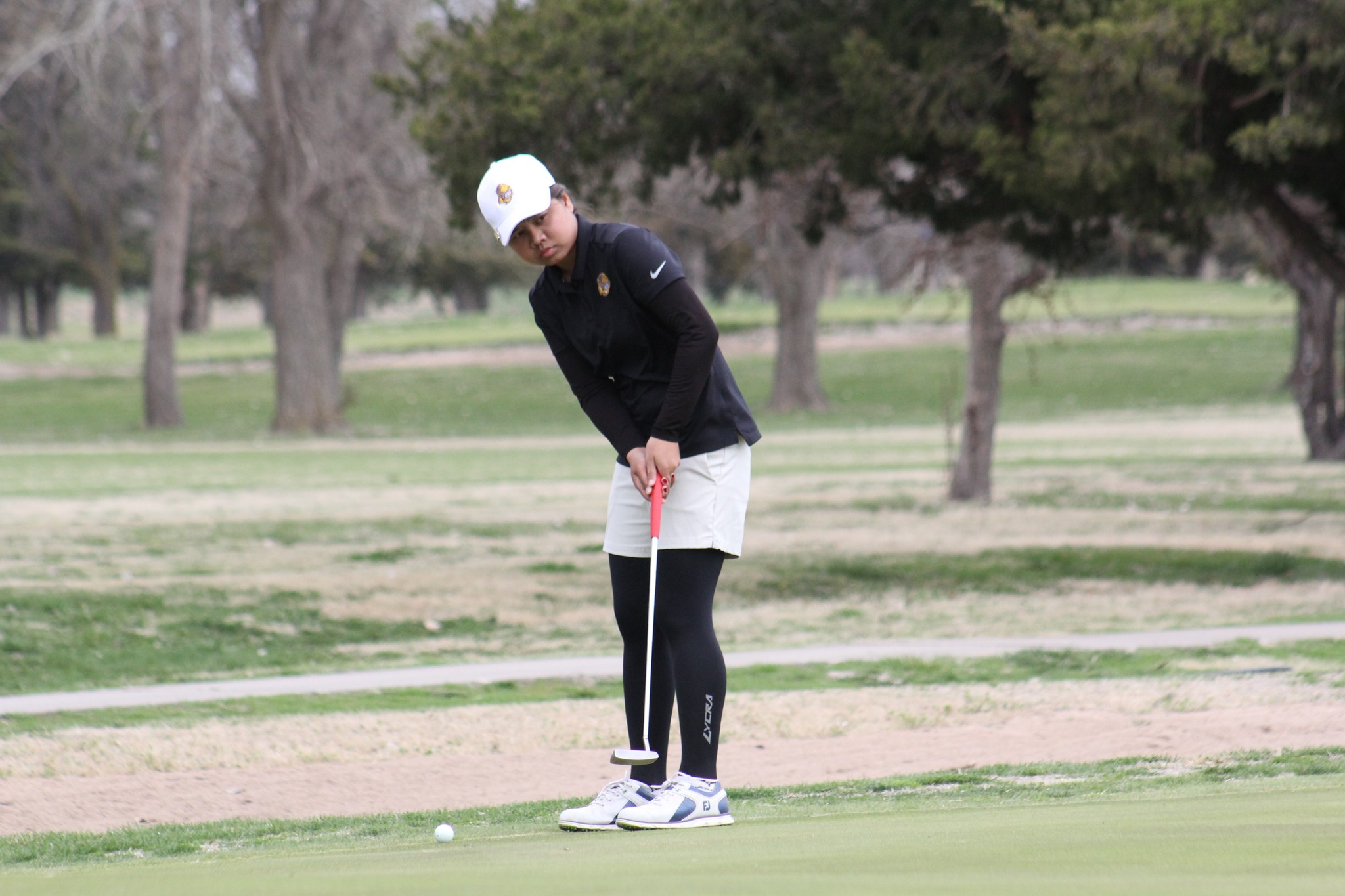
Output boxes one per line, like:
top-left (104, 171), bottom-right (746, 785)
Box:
top-left (610, 465), bottom-right (664, 767)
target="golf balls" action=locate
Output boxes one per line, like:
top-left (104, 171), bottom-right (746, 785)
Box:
top-left (434, 824), bottom-right (455, 843)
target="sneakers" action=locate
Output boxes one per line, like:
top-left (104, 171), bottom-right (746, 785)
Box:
top-left (558, 778), bottom-right (662, 832)
top-left (616, 771), bottom-right (735, 830)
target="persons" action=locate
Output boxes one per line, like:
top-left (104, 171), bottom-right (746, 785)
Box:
top-left (478, 153), bottom-right (761, 829)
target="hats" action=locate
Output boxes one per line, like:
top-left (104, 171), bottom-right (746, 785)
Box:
top-left (477, 153), bottom-right (556, 247)
